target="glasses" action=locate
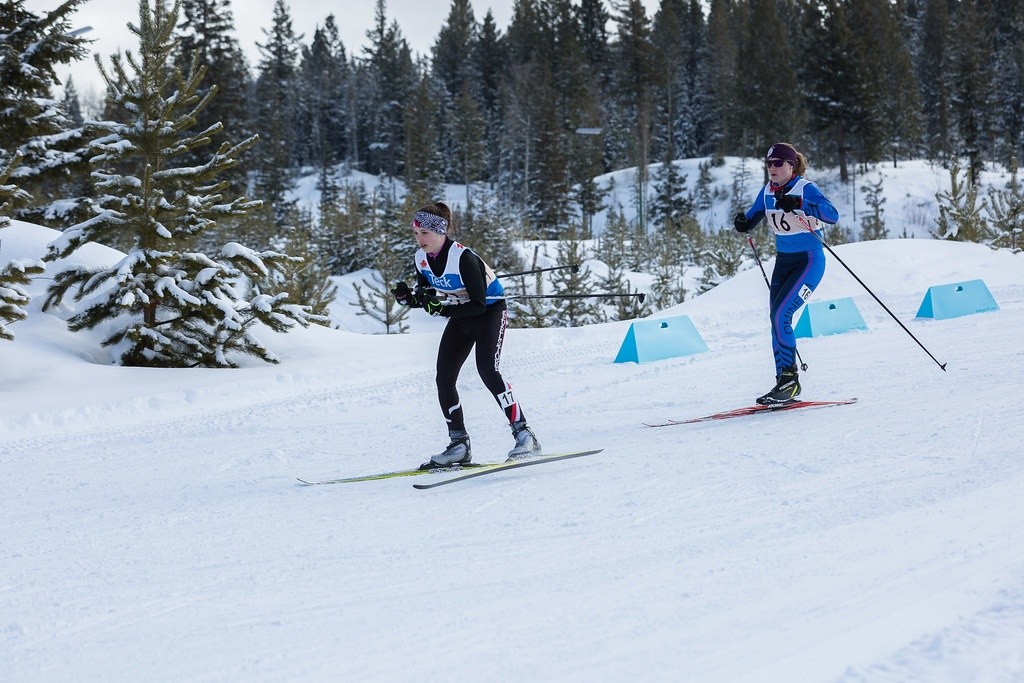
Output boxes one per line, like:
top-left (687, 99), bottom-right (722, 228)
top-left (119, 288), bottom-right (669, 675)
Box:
top-left (765, 160), bottom-right (794, 168)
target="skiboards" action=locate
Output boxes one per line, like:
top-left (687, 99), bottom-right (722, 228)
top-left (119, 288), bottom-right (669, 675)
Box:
top-left (641, 397), bottom-right (859, 427)
top-left (296, 449), bottom-right (604, 490)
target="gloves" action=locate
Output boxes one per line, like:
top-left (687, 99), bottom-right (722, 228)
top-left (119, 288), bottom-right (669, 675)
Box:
top-left (394, 282), bottom-right (417, 307)
top-left (734, 213), bottom-right (751, 232)
top-left (775, 195), bottom-right (801, 213)
top-left (416, 290), bottom-right (445, 317)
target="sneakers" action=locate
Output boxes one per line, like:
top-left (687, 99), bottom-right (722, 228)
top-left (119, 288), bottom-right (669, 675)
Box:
top-left (757, 365), bottom-right (801, 404)
top-left (431, 432), bottom-right (472, 465)
top-left (509, 422), bottom-right (541, 457)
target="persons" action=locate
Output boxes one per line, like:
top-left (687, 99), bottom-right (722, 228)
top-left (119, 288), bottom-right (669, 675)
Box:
top-left (734, 143), bottom-right (839, 405)
top-left (391, 202), bottom-right (542, 467)
